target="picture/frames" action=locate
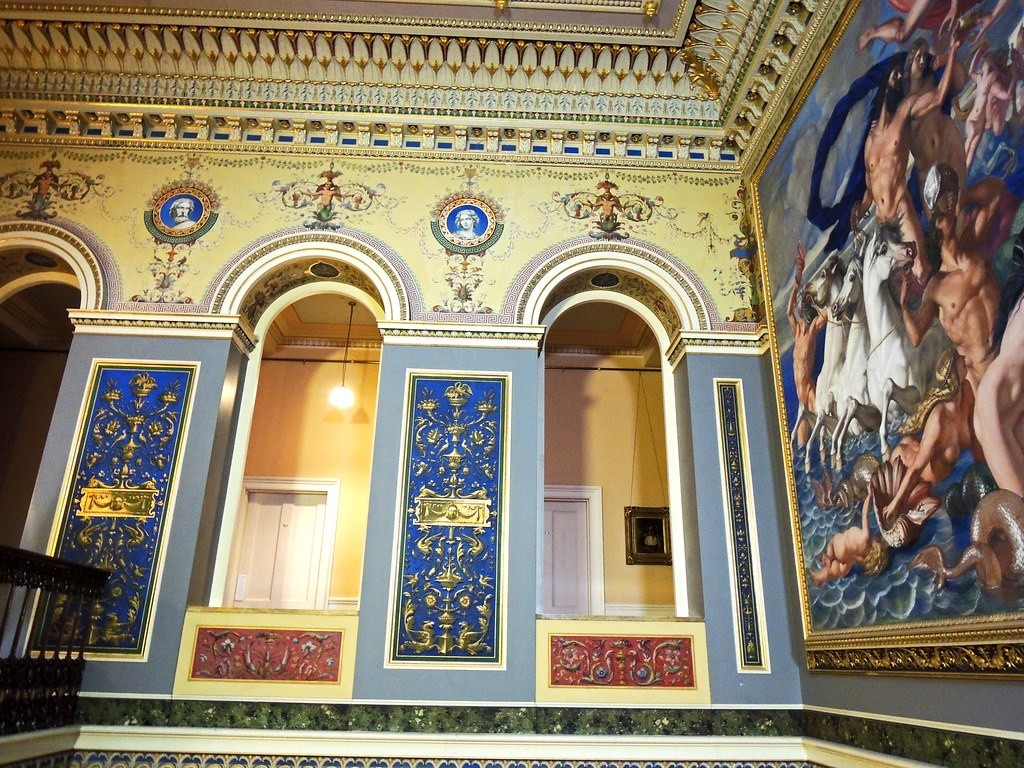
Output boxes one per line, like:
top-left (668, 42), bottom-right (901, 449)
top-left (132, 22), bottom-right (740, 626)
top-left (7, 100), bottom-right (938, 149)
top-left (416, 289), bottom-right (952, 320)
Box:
top-left (739, 0), bottom-right (1024, 682)
top-left (624, 506), bottom-right (672, 566)
